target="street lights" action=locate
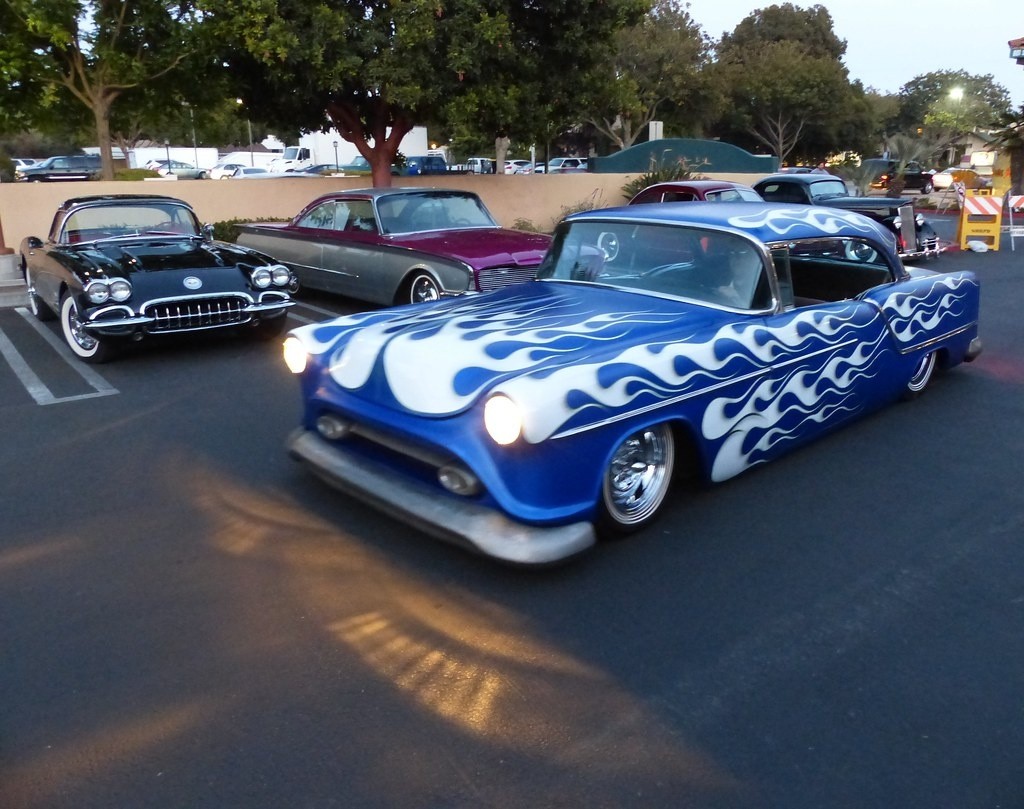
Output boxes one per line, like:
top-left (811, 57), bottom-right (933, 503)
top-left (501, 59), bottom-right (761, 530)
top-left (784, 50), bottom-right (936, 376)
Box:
top-left (164, 138), bottom-right (174, 175)
top-left (948, 86), bottom-right (961, 168)
top-left (333, 141), bottom-right (339, 173)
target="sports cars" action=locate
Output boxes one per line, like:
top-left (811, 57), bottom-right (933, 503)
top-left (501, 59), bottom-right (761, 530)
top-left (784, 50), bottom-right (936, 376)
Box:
top-left (283, 203), bottom-right (982, 565)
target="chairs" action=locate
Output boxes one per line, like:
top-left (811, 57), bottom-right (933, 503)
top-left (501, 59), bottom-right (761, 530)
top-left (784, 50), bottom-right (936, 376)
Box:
top-left (774, 182), bottom-right (805, 203)
top-left (409, 206), bottom-right (450, 232)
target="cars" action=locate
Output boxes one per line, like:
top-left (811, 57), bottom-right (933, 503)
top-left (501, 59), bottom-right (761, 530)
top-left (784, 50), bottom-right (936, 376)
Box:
top-left (10, 159), bottom-right (37, 178)
top-left (406, 156), bottom-right (446, 176)
top-left (341, 156), bottom-right (409, 177)
top-left (207, 163), bottom-right (245, 180)
top-left (516, 163), bottom-right (546, 174)
top-left (751, 174), bottom-right (936, 253)
top-left (233, 167), bottom-right (270, 178)
top-left (18, 155), bottom-right (104, 182)
top-left (567, 181), bottom-right (765, 264)
top-left (548, 157), bottom-right (588, 173)
top-left (296, 164), bottom-right (339, 174)
top-left (466, 158), bottom-right (493, 174)
top-left (228, 188), bottom-right (554, 309)
top-left (933, 167), bottom-right (974, 189)
top-left (779, 168), bottom-right (813, 174)
top-left (145, 159), bottom-right (210, 180)
top-left (858, 159), bottom-right (933, 194)
top-left (504, 160), bottom-right (530, 175)
top-left (19, 194), bottom-right (298, 364)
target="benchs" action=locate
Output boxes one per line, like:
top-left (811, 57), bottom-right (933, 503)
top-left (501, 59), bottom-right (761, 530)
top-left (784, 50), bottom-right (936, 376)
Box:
top-left (753, 255), bottom-right (891, 310)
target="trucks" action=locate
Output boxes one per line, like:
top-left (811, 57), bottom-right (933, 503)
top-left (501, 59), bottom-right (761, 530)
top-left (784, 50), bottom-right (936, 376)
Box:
top-left (272, 126), bottom-right (427, 175)
